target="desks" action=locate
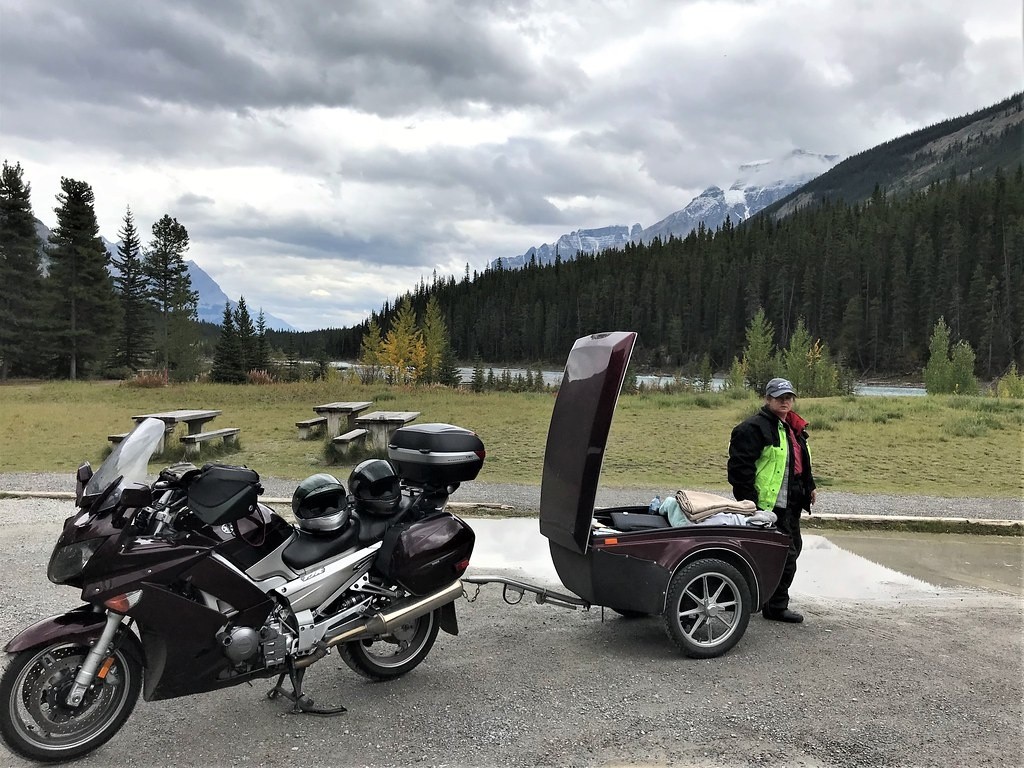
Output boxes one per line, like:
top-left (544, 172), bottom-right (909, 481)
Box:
top-left (313, 401), bottom-right (371, 438)
top-left (127, 409), bottom-right (222, 459)
top-left (355, 411), bottom-right (422, 454)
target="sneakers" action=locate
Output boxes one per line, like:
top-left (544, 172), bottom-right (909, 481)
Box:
top-left (762, 609), bottom-right (803, 622)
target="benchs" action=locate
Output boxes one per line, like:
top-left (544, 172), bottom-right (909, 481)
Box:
top-left (108, 426), bottom-right (174, 457)
top-left (180, 426), bottom-right (242, 460)
top-left (332, 428), bottom-right (369, 454)
top-left (295, 416), bottom-right (327, 439)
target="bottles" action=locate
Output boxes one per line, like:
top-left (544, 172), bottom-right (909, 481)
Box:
top-left (649, 495), bottom-right (661, 515)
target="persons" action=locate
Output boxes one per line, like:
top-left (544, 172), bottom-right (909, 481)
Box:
top-left (727, 378), bottom-right (817, 623)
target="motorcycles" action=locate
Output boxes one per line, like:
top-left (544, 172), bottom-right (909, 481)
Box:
top-left (0, 419), bottom-right (486, 761)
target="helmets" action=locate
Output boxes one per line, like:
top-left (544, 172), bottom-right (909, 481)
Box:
top-left (348, 459), bottom-right (403, 514)
top-left (292, 473), bottom-right (350, 532)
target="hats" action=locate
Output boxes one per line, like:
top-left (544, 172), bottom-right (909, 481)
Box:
top-left (766, 378), bottom-right (798, 398)
